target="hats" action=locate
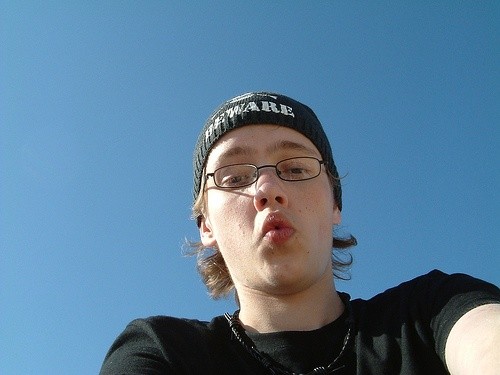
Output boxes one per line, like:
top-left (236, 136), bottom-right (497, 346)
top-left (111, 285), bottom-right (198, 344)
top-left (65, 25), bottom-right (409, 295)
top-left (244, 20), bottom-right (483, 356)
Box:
top-left (192, 90), bottom-right (342, 229)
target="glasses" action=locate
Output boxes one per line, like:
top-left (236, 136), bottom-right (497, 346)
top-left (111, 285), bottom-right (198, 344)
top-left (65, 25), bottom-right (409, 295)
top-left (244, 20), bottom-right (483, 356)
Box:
top-left (203, 155), bottom-right (325, 189)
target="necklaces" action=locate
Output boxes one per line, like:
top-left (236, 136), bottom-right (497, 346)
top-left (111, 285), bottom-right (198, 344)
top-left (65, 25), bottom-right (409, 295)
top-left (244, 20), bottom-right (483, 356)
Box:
top-left (223, 290), bottom-right (356, 375)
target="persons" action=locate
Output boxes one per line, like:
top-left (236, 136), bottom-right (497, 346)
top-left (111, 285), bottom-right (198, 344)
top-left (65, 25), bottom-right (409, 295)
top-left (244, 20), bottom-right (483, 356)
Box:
top-left (96, 92), bottom-right (500, 375)
